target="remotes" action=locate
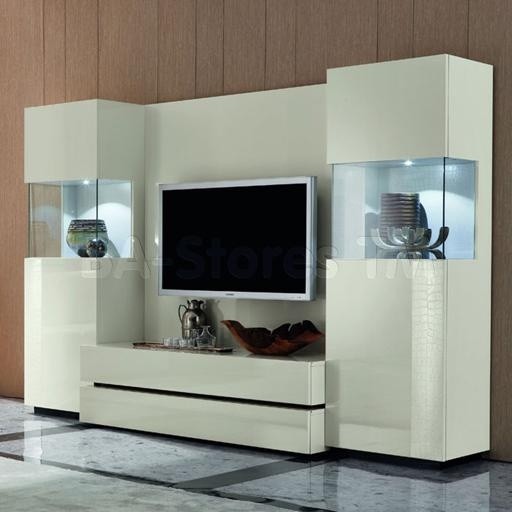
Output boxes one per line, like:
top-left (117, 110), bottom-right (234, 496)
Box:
top-left (207, 346), bottom-right (233, 352)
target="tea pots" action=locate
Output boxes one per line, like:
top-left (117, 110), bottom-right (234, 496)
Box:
top-left (177, 299), bottom-right (208, 340)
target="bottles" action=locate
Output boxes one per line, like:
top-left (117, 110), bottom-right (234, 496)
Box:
top-left (196, 326), bottom-right (216, 347)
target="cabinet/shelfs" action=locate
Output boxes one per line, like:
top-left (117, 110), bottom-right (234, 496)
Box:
top-left (22, 99), bottom-right (143, 415)
top-left (79, 345), bottom-right (325, 454)
top-left (325, 54), bottom-right (493, 462)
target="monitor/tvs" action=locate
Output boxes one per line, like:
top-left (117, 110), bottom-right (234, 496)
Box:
top-left (156, 176), bottom-right (318, 302)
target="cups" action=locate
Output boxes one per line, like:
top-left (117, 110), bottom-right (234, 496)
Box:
top-left (180, 336), bottom-right (188, 349)
top-left (164, 337), bottom-right (173, 345)
top-left (173, 336), bottom-right (181, 345)
